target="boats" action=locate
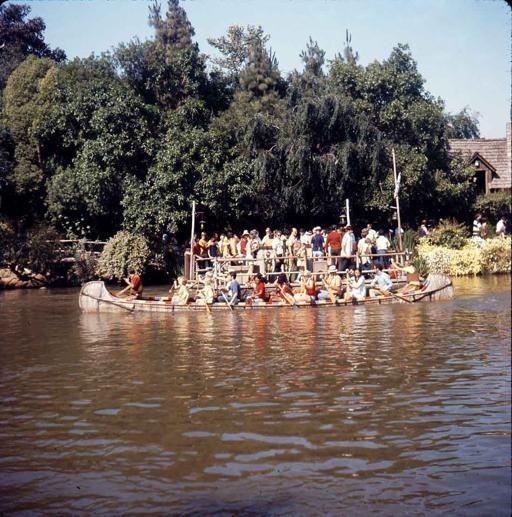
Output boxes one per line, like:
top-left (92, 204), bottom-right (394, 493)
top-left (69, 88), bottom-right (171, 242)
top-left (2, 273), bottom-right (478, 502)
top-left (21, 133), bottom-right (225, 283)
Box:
top-left (79, 272), bottom-right (452, 311)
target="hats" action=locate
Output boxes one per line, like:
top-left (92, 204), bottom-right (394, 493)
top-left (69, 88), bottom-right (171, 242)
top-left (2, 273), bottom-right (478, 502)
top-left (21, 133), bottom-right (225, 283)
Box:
top-left (242, 230), bottom-right (249, 236)
top-left (328, 266), bottom-right (338, 272)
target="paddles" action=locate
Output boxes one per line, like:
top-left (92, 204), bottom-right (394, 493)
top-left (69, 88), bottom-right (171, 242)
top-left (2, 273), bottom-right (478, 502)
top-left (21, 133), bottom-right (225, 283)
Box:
top-left (373, 286), bottom-right (398, 293)
top-left (346, 268), bottom-right (351, 301)
top-left (277, 285), bottom-right (295, 304)
top-left (300, 275), bottom-right (316, 307)
top-left (322, 279), bottom-right (337, 303)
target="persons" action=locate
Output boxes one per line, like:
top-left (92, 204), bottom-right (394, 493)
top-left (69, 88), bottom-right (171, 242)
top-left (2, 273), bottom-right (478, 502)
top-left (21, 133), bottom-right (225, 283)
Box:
top-left (472, 213), bottom-right (481, 238)
top-left (170, 219), bottom-right (428, 305)
top-left (119, 266), bottom-right (144, 300)
top-left (496, 215), bottom-right (509, 237)
